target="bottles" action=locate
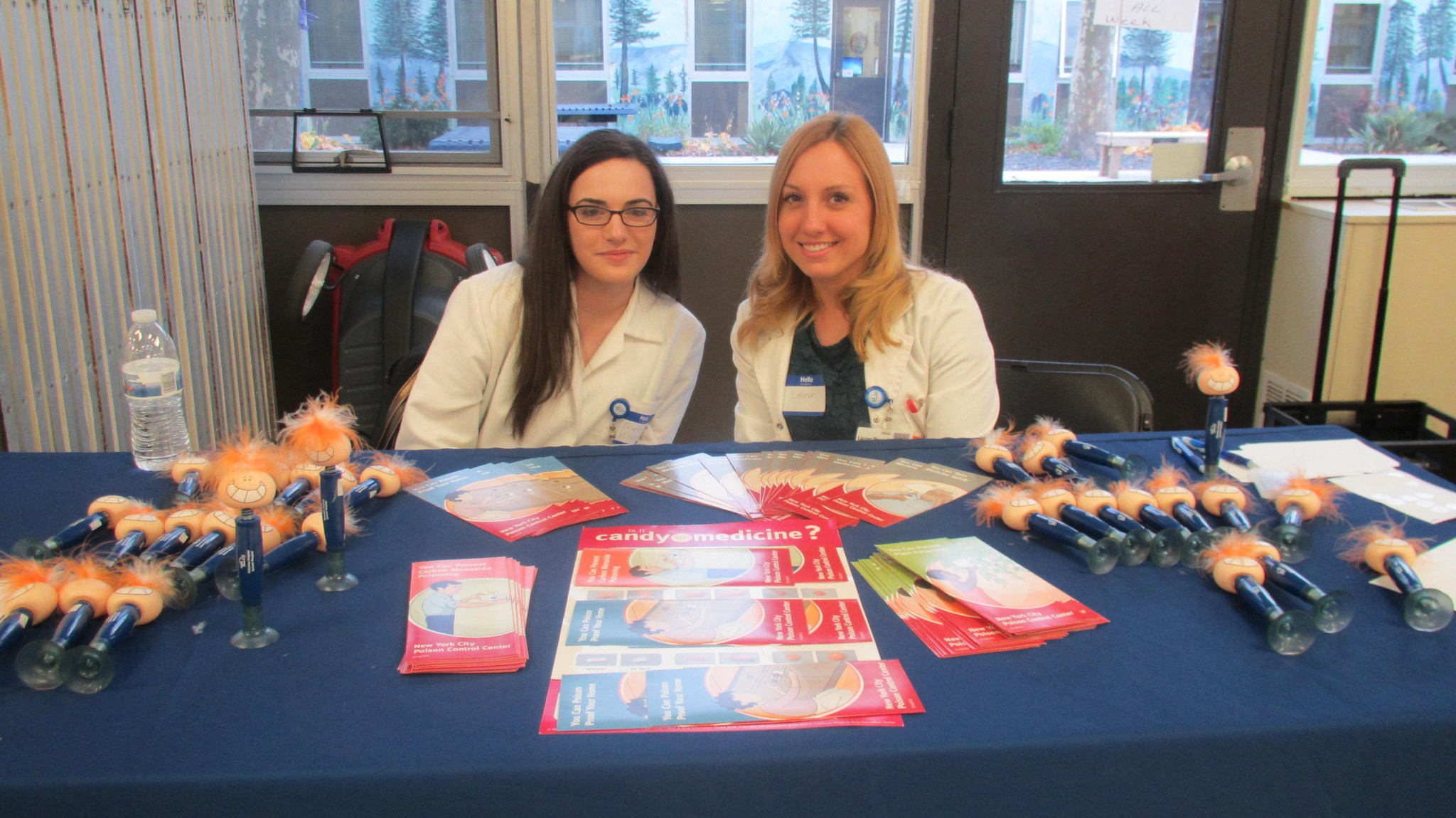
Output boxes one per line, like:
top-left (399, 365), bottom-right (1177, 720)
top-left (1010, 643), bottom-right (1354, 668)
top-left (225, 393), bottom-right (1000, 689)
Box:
top-left (118, 309), bottom-right (193, 472)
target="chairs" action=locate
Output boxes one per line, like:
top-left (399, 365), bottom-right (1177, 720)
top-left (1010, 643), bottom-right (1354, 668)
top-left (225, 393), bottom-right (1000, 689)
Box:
top-left (995, 359), bottom-right (1158, 433)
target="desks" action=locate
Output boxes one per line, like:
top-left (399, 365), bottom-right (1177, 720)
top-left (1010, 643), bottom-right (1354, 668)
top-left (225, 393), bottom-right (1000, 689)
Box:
top-left (0, 422), bottom-right (1456, 817)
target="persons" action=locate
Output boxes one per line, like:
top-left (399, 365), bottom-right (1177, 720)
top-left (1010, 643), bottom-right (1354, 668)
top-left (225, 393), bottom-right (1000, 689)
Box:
top-left (394, 128), bottom-right (707, 451)
top-left (730, 109), bottom-right (1001, 443)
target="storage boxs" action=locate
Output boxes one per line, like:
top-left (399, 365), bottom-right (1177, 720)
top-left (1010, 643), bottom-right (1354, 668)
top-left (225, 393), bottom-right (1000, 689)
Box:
top-left (1262, 399), bottom-right (1456, 484)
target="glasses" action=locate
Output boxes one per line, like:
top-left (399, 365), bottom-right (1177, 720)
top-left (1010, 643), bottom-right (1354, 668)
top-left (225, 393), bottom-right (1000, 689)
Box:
top-left (565, 203), bottom-right (661, 227)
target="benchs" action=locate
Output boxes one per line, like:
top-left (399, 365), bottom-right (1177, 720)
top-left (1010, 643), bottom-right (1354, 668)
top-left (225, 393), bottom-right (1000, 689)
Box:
top-left (1095, 130), bottom-right (1210, 181)
